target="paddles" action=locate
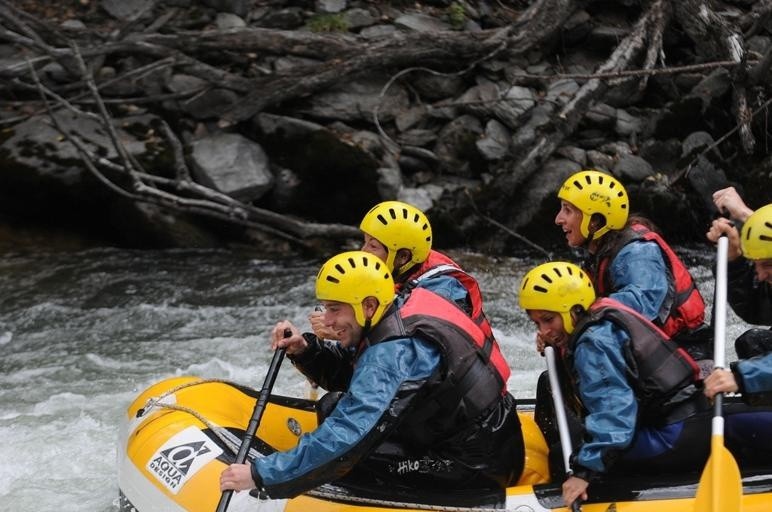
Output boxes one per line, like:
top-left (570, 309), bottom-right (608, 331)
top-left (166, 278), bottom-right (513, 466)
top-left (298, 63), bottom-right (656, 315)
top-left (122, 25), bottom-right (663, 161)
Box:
top-left (692, 205), bottom-right (745, 512)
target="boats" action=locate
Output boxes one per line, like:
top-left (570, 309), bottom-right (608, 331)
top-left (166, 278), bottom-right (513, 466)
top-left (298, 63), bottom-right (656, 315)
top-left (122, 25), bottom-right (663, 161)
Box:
top-left (117, 377), bottom-right (772, 512)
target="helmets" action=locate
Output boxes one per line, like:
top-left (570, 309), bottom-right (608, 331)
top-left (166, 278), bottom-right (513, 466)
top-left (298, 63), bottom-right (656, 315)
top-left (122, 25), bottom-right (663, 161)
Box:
top-left (557, 170), bottom-right (631, 240)
top-left (360, 195), bottom-right (435, 273)
top-left (314, 249), bottom-right (393, 328)
top-left (738, 202), bottom-right (772, 261)
top-left (517, 262), bottom-right (596, 335)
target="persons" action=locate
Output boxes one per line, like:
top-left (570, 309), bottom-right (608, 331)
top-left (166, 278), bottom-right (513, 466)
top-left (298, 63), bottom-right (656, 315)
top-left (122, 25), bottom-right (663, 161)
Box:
top-left (221, 252), bottom-right (525, 509)
top-left (553, 172), bottom-right (708, 367)
top-left (704, 177), bottom-right (772, 411)
top-left (517, 261), bottom-right (711, 507)
top-left (315, 200), bottom-right (510, 449)
